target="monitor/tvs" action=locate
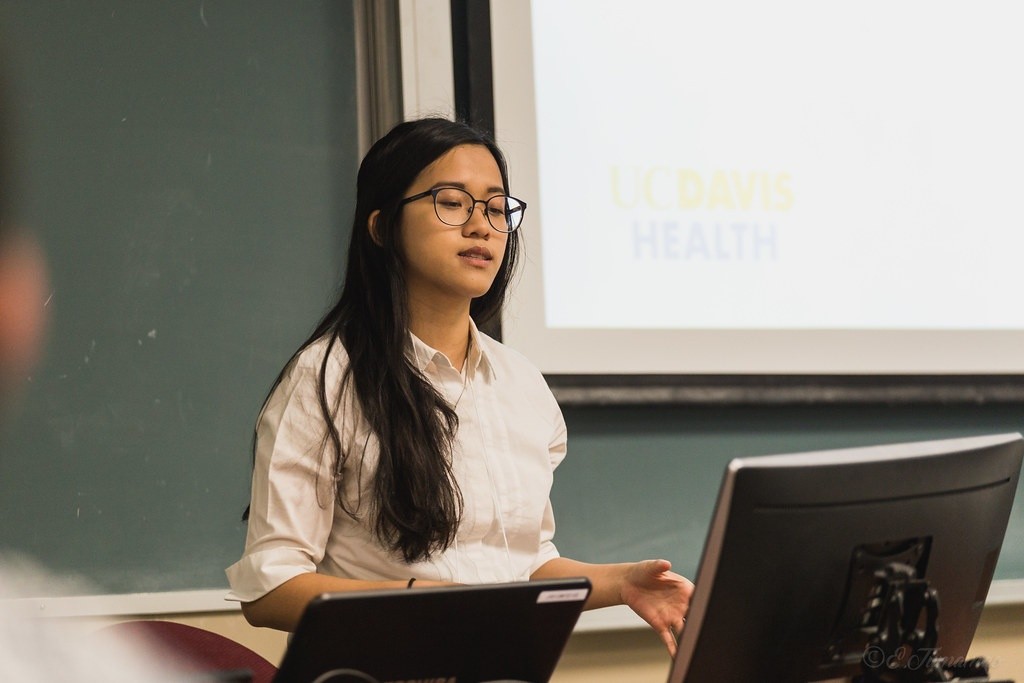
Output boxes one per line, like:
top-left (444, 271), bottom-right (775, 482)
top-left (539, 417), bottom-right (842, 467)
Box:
top-left (661, 431), bottom-right (1024, 683)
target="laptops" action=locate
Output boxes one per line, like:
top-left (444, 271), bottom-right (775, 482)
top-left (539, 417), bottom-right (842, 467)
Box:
top-left (273, 577), bottom-right (592, 683)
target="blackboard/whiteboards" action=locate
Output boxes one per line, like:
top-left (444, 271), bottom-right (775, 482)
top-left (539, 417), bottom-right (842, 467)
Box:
top-left (0, 0), bottom-right (1024, 635)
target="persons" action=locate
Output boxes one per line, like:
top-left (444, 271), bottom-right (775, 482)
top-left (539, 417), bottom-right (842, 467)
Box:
top-left (226, 115), bottom-right (702, 664)
top-left (0, 124), bottom-right (219, 680)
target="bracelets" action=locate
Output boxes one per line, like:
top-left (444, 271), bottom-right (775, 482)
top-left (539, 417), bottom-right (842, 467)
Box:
top-left (406, 577), bottom-right (417, 588)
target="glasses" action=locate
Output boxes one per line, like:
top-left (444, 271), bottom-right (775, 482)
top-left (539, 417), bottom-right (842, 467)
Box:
top-left (401, 187), bottom-right (526, 234)
